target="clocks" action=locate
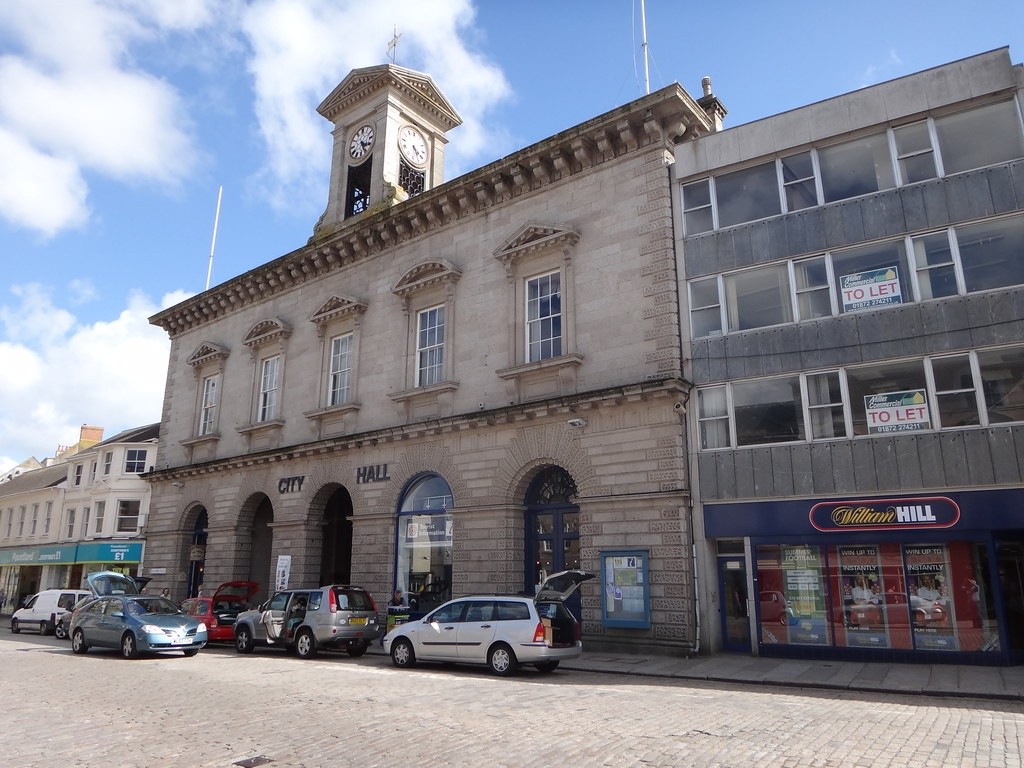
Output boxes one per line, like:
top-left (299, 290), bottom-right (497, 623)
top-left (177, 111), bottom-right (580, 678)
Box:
top-left (350, 127), bottom-right (373, 158)
top-left (401, 129), bottom-right (428, 165)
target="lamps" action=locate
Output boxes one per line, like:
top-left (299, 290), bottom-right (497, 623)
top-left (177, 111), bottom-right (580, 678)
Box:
top-left (568, 418), bottom-right (586, 428)
top-left (173, 483), bottom-right (183, 486)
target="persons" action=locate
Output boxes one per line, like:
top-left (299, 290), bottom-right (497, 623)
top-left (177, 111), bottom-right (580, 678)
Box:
top-left (128, 602), bottom-right (141, 615)
top-left (380, 590), bottom-right (406, 645)
top-left (888, 583), bottom-right (897, 592)
top-left (160, 588), bottom-right (172, 613)
top-left (198, 585), bottom-right (202, 598)
top-left (151, 600), bottom-right (164, 613)
top-left (854, 575), bottom-right (879, 606)
top-left (893, 594), bottom-right (900, 604)
top-left (919, 575), bottom-right (946, 606)
top-left (0, 589), bottom-right (6, 608)
top-left (139, 586), bottom-right (151, 612)
top-left (963, 579), bottom-right (986, 628)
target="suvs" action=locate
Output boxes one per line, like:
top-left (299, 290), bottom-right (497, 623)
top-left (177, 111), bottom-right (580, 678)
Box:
top-left (234, 583), bottom-right (380, 658)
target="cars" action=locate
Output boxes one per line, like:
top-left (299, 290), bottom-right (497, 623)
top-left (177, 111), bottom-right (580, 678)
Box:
top-left (9, 588), bottom-right (91, 636)
top-left (825, 592), bottom-right (947, 630)
top-left (54, 577), bottom-right (153, 640)
top-left (180, 579), bottom-right (260, 643)
top-left (759, 589), bottom-right (801, 626)
top-left (382, 567), bottom-right (595, 677)
top-left (69, 572), bottom-right (208, 661)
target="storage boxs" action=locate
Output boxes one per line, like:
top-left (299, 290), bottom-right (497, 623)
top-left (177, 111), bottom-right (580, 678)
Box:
top-left (541, 617), bottom-right (561, 646)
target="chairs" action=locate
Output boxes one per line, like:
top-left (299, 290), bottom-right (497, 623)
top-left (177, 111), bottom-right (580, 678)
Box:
top-left (471, 609), bottom-right (482, 621)
top-left (217, 605), bottom-right (224, 610)
